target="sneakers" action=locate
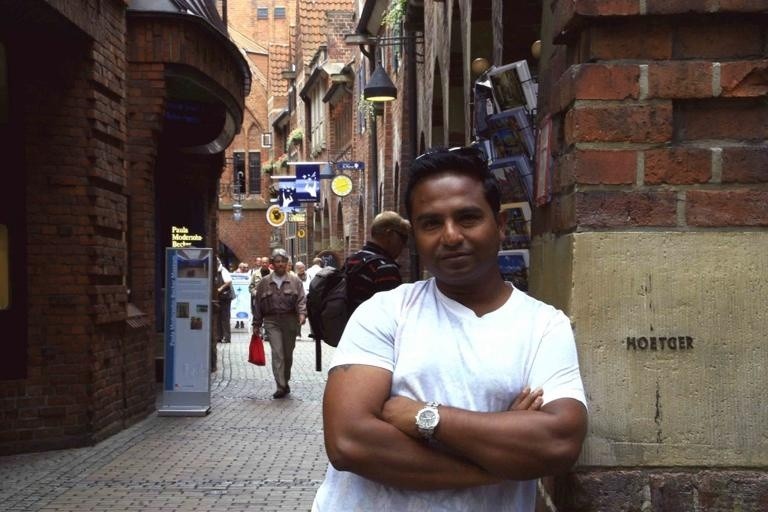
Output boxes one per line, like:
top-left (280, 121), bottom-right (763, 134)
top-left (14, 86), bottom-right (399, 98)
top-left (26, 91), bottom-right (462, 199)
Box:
top-left (273, 385), bottom-right (290, 398)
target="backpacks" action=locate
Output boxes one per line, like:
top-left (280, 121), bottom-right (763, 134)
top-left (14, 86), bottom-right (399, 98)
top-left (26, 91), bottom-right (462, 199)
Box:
top-left (305, 254), bottom-right (401, 347)
top-left (247, 332), bottom-right (265, 366)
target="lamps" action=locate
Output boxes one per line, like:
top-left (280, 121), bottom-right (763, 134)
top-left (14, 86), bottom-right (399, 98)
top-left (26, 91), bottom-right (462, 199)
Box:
top-left (216, 171), bottom-right (246, 223)
top-left (361, 29), bottom-right (426, 102)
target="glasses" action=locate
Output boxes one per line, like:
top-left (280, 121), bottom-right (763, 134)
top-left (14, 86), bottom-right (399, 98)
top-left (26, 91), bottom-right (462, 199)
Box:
top-left (415, 146), bottom-right (489, 168)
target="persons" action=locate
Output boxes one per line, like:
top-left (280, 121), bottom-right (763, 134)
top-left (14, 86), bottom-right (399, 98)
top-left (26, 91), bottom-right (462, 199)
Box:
top-left (339, 209), bottom-right (411, 319)
top-left (309, 150), bottom-right (588, 512)
top-left (215, 247), bottom-right (326, 398)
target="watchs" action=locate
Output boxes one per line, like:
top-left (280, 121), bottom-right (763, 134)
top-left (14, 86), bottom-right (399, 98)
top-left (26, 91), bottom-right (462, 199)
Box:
top-left (415, 401), bottom-right (442, 445)
